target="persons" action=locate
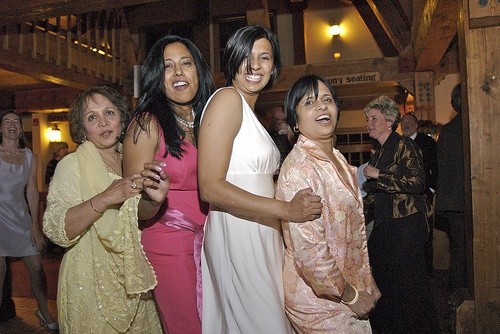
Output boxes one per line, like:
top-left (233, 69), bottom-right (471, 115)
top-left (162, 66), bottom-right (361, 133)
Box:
top-left (124, 35), bottom-right (216, 334)
top-left (276, 75), bottom-right (381, 334)
top-left (46, 142), bottom-right (68, 255)
top-left (363, 95), bottom-right (426, 334)
top-left (397, 112), bottom-right (437, 197)
top-left (435, 83), bottom-right (475, 307)
top-left (264, 106), bottom-right (295, 149)
top-left (0, 111), bottom-right (59, 330)
top-left (197, 25), bottom-right (323, 334)
top-left (43, 86), bottom-right (166, 334)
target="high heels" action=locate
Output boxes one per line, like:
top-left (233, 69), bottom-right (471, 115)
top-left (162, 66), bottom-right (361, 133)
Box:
top-left (35, 306), bottom-right (59, 331)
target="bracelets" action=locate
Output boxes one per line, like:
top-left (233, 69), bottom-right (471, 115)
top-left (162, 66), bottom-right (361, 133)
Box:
top-left (341, 284), bottom-right (359, 305)
top-left (90, 198), bottom-right (104, 214)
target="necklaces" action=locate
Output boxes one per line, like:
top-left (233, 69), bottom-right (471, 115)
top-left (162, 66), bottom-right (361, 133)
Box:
top-left (98, 150), bottom-right (123, 177)
top-left (173, 107), bottom-right (196, 128)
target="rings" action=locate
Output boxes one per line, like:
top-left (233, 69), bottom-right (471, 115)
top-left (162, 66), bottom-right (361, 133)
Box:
top-left (132, 183), bottom-right (137, 189)
